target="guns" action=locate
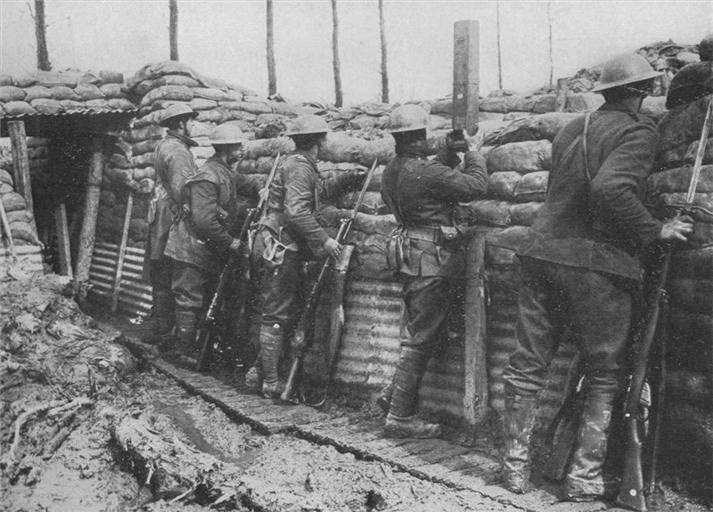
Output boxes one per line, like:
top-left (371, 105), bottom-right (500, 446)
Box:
top-left (279, 159), bottom-right (381, 407)
top-left (193, 154), bottom-right (278, 371)
top-left (614, 97), bottom-right (713, 511)
top-left (543, 362), bottom-right (588, 483)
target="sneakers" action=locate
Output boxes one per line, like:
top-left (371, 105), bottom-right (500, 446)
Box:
top-left (375, 395), bottom-right (390, 411)
top-left (384, 416), bottom-right (442, 439)
top-left (245, 367), bottom-right (286, 399)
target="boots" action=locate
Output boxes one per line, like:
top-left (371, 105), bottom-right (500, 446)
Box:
top-left (563, 396), bottom-right (622, 501)
top-left (502, 397), bottom-right (538, 494)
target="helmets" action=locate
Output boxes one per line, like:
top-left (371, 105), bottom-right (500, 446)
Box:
top-left (592, 52), bottom-right (665, 92)
top-left (387, 104), bottom-right (430, 134)
top-left (159, 103), bottom-right (198, 126)
top-left (286, 114), bottom-right (330, 135)
top-left (209, 125), bottom-right (246, 144)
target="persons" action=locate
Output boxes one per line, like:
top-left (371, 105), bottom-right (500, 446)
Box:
top-left (374, 101), bottom-right (488, 440)
top-left (130, 101), bottom-right (200, 349)
top-left (240, 112), bottom-right (368, 399)
top-left (160, 122), bottom-right (266, 370)
top-left (497, 50), bottom-right (695, 501)
